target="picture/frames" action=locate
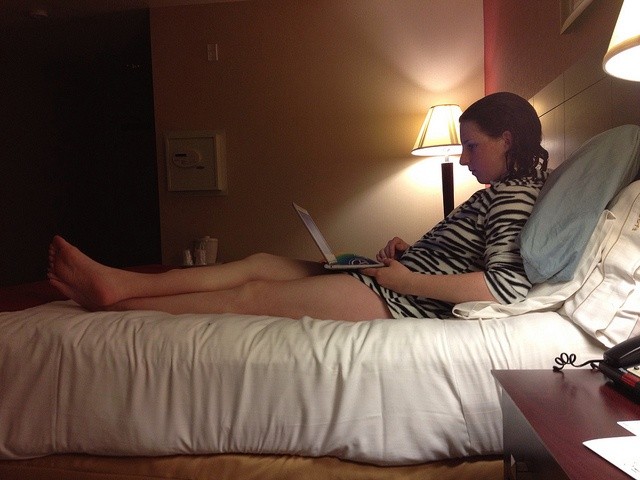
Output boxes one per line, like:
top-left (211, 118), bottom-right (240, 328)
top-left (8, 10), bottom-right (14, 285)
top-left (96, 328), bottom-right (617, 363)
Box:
top-left (557, 1), bottom-right (599, 37)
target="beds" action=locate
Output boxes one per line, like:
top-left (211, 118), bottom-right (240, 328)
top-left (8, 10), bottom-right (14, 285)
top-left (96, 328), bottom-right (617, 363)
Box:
top-left (1, 178), bottom-right (640, 479)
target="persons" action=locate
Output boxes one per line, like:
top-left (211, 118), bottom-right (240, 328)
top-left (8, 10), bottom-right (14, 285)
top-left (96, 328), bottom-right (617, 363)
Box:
top-left (46, 91), bottom-right (556, 322)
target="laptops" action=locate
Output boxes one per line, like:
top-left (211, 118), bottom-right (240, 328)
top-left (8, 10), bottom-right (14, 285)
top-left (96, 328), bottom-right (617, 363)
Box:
top-left (292, 203), bottom-right (385, 270)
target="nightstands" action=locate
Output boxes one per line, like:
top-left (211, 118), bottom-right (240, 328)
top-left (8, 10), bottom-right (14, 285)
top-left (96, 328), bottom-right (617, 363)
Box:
top-left (491, 367), bottom-right (640, 478)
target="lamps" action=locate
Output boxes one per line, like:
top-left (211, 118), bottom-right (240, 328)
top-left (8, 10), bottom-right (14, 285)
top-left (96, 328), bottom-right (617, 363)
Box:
top-left (411, 102), bottom-right (464, 219)
top-left (602, 1), bottom-right (640, 82)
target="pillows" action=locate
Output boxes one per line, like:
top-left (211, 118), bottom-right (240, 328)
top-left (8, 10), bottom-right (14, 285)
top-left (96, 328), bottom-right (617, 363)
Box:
top-left (517, 122), bottom-right (640, 285)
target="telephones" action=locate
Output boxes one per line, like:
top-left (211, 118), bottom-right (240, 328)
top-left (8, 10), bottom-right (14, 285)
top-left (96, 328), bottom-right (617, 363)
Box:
top-left (553, 334), bottom-right (640, 391)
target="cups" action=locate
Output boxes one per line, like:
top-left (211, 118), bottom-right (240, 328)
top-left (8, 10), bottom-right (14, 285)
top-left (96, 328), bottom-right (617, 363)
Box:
top-left (199, 237), bottom-right (221, 263)
